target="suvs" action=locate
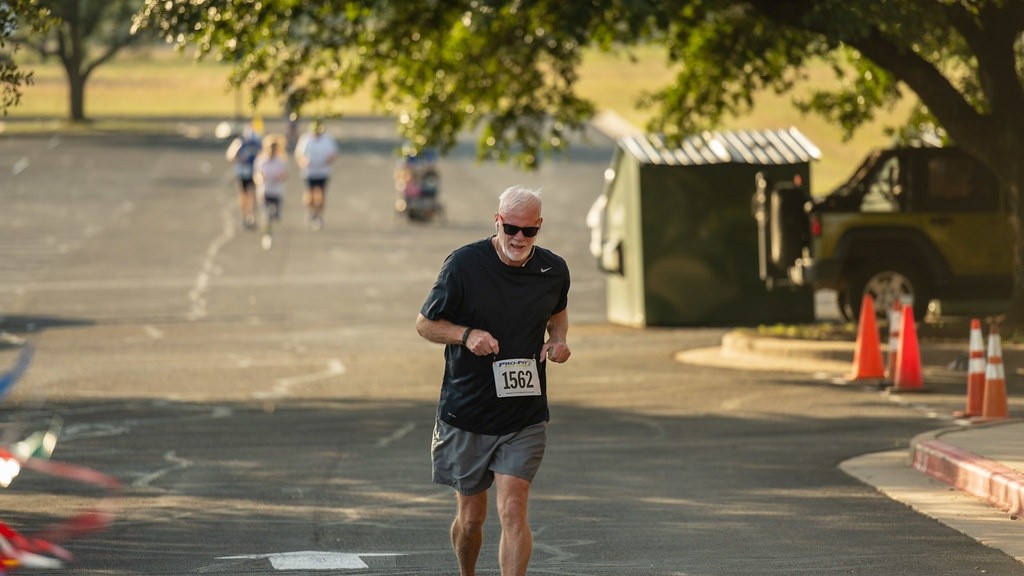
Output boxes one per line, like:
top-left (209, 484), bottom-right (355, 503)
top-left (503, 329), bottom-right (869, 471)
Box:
top-left (807, 144), bottom-right (1024, 323)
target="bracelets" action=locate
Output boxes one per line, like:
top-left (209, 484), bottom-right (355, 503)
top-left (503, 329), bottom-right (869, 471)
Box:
top-left (461, 327), bottom-right (474, 347)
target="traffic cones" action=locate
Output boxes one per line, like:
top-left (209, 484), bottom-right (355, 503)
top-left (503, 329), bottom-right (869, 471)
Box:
top-left (953, 319), bottom-right (988, 418)
top-left (849, 296), bottom-right (887, 388)
top-left (966, 323), bottom-right (1008, 423)
top-left (884, 297), bottom-right (927, 393)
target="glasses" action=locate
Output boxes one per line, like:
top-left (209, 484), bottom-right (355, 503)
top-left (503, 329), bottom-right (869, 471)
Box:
top-left (500, 215), bottom-right (541, 237)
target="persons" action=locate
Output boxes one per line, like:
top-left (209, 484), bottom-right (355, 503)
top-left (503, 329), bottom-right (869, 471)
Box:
top-left (226, 122), bottom-right (264, 231)
top-left (395, 140), bottom-right (441, 220)
top-left (251, 133), bottom-right (289, 250)
top-left (294, 119), bottom-right (338, 231)
top-left (416, 185), bottom-right (573, 576)
